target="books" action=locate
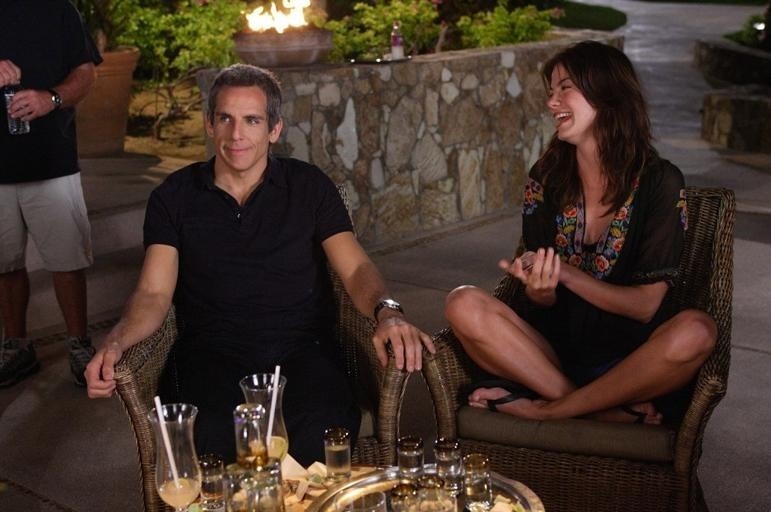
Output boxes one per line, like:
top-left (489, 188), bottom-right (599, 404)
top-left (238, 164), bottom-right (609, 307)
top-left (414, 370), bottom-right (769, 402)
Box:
top-left (373, 298), bottom-right (404, 318)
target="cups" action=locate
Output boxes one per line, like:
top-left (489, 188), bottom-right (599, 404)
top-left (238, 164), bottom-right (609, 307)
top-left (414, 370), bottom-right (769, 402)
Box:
top-left (193, 454), bottom-right (226, 508)
top-left (322, 429), bottom-right (351, 480)
top-left (394, 435), bottom-right (426, 482)
top-left (433, 437), bottom-right (464, 490)
top-left (460, 454), bottom-right (495, 511)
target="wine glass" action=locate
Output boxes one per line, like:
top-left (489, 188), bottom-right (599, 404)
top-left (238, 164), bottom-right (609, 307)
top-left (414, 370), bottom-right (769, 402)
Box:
top-left (144, 403), bottom-right (202, 511)
top-left (239, 374), bottom-right (301, 496)
top-left (344, 488), bottom-right (385, 510)
top-left (219, 470), bottom-right (253, 511)
top-left (247, 469), bottom-right (286, 508)
top-left (231, 407), bottom-right (268, 467)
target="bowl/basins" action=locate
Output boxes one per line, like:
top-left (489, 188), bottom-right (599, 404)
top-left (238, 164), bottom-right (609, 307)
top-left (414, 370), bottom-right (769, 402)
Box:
top-left (305, 462), bottom-right (546, 511)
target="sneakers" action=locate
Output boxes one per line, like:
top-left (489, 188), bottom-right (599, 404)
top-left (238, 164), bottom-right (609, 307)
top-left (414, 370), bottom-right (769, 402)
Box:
top-left (66, 335), bottom-right (97, 387)
top-left (0, 338), bottom-right (40, 391)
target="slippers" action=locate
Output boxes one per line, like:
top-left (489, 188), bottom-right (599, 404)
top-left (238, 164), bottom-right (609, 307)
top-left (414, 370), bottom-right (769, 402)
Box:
top-left (624, 400), bottom-right (669, 426)
top-left (470, 376), bottom-right (531, 415)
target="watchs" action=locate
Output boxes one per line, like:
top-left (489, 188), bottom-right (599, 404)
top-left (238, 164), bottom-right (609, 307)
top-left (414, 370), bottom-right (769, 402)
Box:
top-left (46, 88), bottom-right (63, 111)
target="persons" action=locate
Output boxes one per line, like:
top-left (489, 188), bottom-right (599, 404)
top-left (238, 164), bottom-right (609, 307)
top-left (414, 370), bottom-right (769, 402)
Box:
top-left (84, 63), bottom-right (435, 466)
top-left (1, 0), bottom-right (105, 388)
top-left (443, 39), bottom-right (719, 428)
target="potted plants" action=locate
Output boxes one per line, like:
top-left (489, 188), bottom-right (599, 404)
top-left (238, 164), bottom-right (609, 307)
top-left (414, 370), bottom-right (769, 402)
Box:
top-left (63, 0), bottom-right (139, 158)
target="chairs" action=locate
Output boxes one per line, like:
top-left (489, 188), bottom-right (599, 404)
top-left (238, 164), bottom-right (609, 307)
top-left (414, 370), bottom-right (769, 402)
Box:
top-left (107, 260), bottom-right (410, 512)
top-left (420, 186), bottom-right (737, 512)
